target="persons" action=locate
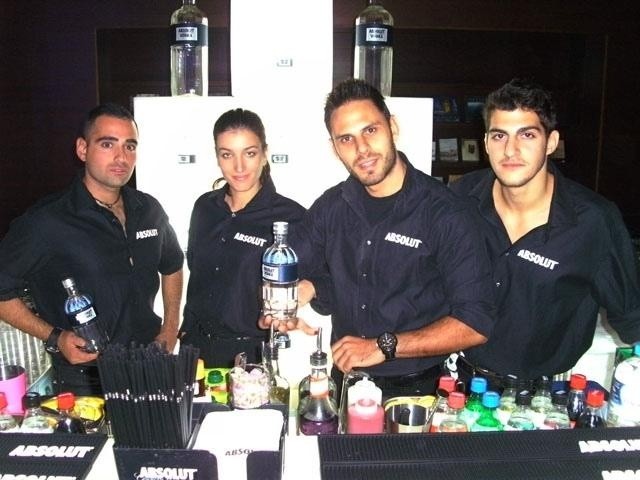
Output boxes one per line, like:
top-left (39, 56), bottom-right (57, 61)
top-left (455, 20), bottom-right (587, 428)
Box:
top-left (269, 78), bottom-right (503, 401)
top-left (442, 78), bottom-right (640, 387)
top-left (0, 102), bottom-right (183, 370)
top-left (177, 108), bottom-right (309, 373)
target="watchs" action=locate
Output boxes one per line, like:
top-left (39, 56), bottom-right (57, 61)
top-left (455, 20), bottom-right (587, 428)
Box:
top-left (378, 330), bottom-right (397, 361)
top-left (44, 325), bottom-right (63, 356)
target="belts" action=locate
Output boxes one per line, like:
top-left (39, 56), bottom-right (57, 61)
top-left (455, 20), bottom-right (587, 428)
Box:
top-left (348, 365), bottom-right (441, 389)
top-left (187, 310), bottom-right (267, 344)
top-left (456, 356), bottom-right (543, 395)
top-left (70, 365), bottom-right (101, 377)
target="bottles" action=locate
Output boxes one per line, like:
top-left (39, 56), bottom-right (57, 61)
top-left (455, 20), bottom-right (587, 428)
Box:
top-left (0, 393), bottom-right (85, 437)
top-left (354, 0), bottom-right (394, 97)
top-left (169, 0), bottom-right (209, 96)
top-left (260, 342), bottom-right (290, 404)
top-left (299, 352), bottom-right (385, 435)
top-left (608, 342), bottom-right (640, 428)
top-left (262, 222), bottom-right (300, 321)
top-left (63, 278), bottom-right (109, 354)
top-left (425, 374), bottom-right (606, 432)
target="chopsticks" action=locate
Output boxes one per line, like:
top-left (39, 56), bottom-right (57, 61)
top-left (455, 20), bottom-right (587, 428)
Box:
top-left (96, 341), bottom-right (199, 449)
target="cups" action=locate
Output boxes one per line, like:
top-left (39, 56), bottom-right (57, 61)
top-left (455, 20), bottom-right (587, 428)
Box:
top-left (225, 363), bottom-right (271, 410)
top-left (385, 404), bottom-right (428, 435)
top-left (0, 366), bottom-right (27, 415)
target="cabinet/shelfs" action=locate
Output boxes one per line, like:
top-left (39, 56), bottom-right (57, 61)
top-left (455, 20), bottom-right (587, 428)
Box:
top-left (433, 84), bottom-right (580, 195)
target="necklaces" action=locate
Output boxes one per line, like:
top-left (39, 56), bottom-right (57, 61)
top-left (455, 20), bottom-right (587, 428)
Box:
top-left (92, 193), bottom-right (122, 209)
